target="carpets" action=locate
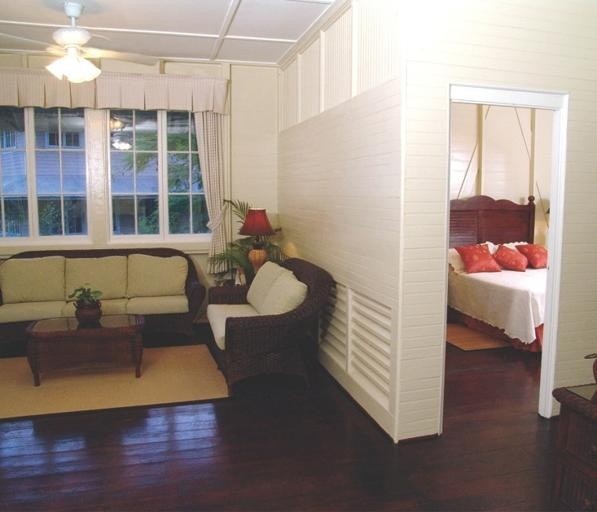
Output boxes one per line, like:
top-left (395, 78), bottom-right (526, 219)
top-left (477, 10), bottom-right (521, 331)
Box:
top-left (446, 323), bottom-right (512, 352)
top-left (0, 342), bottom-right (231, 419)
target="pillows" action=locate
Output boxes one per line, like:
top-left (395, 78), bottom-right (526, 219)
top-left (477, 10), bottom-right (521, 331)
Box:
top-left (448, 241), bottom-right (549, 275)
top-left (260, 271), bottom-right (309, 315)
top-left (244, 261), bottom-right (293, 314)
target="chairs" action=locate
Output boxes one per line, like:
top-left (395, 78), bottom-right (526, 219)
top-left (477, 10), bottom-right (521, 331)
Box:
top-left (204, 258), bottom-right (334, 387)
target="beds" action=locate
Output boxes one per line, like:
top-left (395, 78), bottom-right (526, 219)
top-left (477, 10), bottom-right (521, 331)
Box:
top-left (448, 193), bottom-right (548, 351)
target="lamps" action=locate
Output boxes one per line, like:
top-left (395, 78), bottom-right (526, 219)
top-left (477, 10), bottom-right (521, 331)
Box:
top-left (45, 49), bottom-right (102, 84)
top-left (235, 206), bottom-right (274, 276)
top-left (110, 125), bottom-right (134, 150)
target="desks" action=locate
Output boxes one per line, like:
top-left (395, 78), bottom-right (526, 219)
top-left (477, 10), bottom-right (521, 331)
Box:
top-left (25, 315), bottom-right (147, 387)
top-left (550, 382), bottom-right (596, 512)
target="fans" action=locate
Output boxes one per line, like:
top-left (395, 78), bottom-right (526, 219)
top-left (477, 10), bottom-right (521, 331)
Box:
top-left (0, 1), bottom-right (158, 67)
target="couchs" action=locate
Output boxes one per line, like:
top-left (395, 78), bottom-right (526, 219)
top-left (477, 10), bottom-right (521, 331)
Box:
top-left (0, 245), bottom-right (209, 354)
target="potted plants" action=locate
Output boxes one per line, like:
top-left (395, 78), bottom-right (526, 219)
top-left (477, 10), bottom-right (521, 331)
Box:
top-left (67, 285), bottom-right (103, 324)
